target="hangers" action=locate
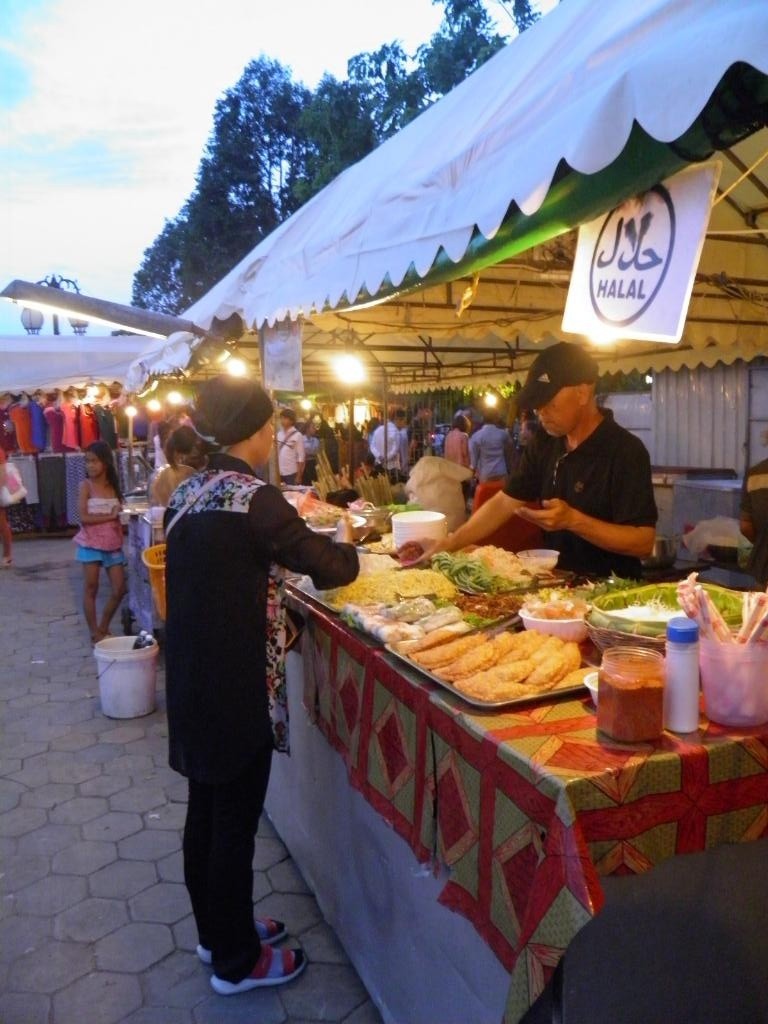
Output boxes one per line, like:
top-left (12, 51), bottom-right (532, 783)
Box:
top-left (7, 446), bottom-right (86, 460)
top-left (0, 383), bottom-right (126, 410)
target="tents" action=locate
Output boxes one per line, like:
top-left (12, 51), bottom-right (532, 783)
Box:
top-left (120, 0), bottom-right (768, 636)
top-left (0, 336), bottom-right (173, 397)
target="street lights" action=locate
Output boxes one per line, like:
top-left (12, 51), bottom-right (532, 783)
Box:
top-left (20, 273), bottom-right (91, 337)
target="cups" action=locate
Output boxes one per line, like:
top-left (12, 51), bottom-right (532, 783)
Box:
top-left (698, 632), bottom-right (768, 726)
top-left (150, 506), bottom-right (166, 520)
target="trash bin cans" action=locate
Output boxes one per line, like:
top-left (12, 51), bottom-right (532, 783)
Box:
top-left (142, 544), bottom-right (166, 620)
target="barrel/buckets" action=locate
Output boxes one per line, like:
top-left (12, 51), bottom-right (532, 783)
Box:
top-left (92, 635), bottom-right (161, 719)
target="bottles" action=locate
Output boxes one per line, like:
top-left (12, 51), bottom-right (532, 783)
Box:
top-left (596, 644), bottom-right (665, 744)
top-left (132, 631), bottom-right (153, 650)
top-left (664, 616), bottom-right (700, 735)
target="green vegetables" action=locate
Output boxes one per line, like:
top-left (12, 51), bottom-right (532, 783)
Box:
top-left (385, 503), bottom-right (422, 511)
top-left (567, 568), bottom-right (642, 602)
top-left (432, 550), bottom-right (539, 594)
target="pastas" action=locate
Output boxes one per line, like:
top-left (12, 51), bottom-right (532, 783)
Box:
top-left (322, 567), bottom-right (459, 606)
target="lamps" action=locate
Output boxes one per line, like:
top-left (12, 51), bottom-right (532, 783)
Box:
top-left (0, 281), bottom-right (252, 365)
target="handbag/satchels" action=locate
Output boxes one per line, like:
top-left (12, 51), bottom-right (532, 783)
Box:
top-left (0, 463), bottom-right (27, 507)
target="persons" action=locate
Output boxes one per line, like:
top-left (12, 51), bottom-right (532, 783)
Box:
top-left (0, 409), bottom-right (28, 567)
top-left (277, 402), bottom-right (552, 486)
top-left (401, 341), bottom-right (658, 583)
top-left (739, 458), bottom-right (768, 587)
top-left (163, 369), bottom-right (360, 993)
top-left (72, 440), bottom-right (127, 649)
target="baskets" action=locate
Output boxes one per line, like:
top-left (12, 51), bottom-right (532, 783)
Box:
top-left (583, 611), bottom-right (741, 657)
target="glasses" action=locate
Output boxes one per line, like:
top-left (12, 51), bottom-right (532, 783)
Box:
top-left (551, 453), bottom-right (568, 489)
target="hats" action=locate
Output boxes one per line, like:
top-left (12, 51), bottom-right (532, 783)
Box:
top-left (193, 373), bottom-right (273, 448)
top-left (509, 342), bottom-right (599, 410)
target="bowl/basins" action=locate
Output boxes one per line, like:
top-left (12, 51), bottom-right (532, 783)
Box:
top-left (516, 548), bottom-right (561, 569)
top-left (518, 608), bottom-right (588, 644)
top-left (583, 673), bottom-right (599, 706)
top-left (125, 496), bottom-right (146, 503)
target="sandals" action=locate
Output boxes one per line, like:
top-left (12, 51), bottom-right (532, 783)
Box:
top-left (209, 942), bottom-right (307, 996)
top-left (196, 917), bottom-right (287, 963)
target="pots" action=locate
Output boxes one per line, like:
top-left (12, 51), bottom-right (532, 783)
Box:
top-left (348, 501), bottom-right (398, 534)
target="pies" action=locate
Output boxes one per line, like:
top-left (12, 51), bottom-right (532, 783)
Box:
top-left (401, 622), bottom-right (594, 702)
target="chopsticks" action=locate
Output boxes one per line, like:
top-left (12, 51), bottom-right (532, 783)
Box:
top-left (675, 576), bottom-right (768, 642)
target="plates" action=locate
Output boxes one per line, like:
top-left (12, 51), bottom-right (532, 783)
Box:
top-left (392, 510), bottom-right (449, 551)
top-left (306, 514), bottom-right (367, 532)
